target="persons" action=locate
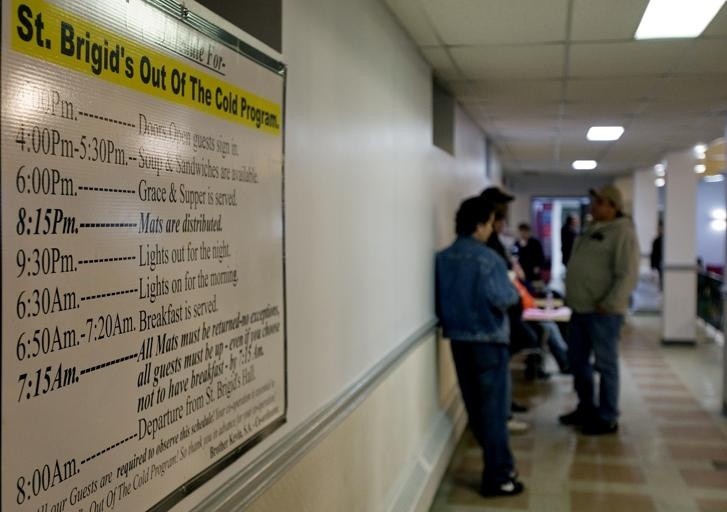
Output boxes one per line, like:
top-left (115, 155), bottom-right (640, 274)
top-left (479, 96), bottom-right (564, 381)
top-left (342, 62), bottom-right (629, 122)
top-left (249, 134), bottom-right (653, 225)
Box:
top-left (651, 221), bottom-right (664, 289)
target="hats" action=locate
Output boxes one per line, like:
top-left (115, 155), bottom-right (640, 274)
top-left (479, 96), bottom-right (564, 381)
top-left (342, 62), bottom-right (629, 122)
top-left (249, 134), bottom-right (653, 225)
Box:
top-left (481, 186), bottom-right (517, 203)
top-left (589, 184), bottom-right (626, 210)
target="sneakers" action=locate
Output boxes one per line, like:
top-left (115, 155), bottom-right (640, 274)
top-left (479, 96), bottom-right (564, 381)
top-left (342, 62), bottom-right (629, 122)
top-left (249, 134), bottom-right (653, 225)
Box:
top-left (558, 407), bottom-right (620, 438)
top-left (504, 420), bottom-right (529, 432)
top-left (477, 480), bottom-right (526, 500)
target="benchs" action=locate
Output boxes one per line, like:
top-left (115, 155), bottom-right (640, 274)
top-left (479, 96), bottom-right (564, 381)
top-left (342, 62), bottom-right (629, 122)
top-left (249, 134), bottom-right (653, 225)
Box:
top-left (696, 270), bottom-right (724, 340)
